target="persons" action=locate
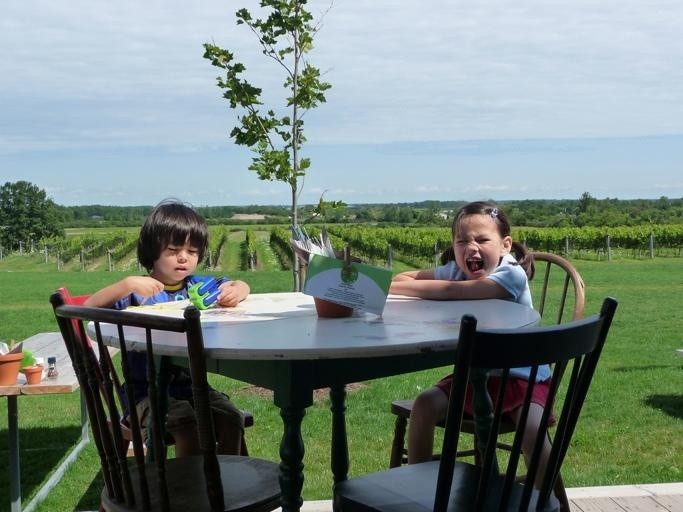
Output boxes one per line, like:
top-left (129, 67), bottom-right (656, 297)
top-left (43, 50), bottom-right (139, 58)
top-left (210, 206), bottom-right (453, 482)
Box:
top-left (84, 199), bottom-right (250, 459)
top-left (382, 201), bottom-right (556, 499)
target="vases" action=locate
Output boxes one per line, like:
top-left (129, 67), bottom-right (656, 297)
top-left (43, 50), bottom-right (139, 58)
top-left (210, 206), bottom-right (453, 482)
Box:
top-left (0, 353), bottom-right (23, 386)
top-left (23, 365), bottom-right (43, 384)
top-left (314, 293), bottom-right (352, 318)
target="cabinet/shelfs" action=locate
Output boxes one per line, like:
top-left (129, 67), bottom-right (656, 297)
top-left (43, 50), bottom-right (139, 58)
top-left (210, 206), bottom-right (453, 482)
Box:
top-left (0, 332), bottom-right (120, 512)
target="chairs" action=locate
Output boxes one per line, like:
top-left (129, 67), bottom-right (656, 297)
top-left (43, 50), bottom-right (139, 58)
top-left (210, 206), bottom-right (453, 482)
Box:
top-left (59, 288), bottom-right (254, 471)
top-left (50, 294), bottom-right (282, 512)
top-left (338, 298), bottom-right (622, 512)
top-left (391, 253), bottom-right (586, 468)
